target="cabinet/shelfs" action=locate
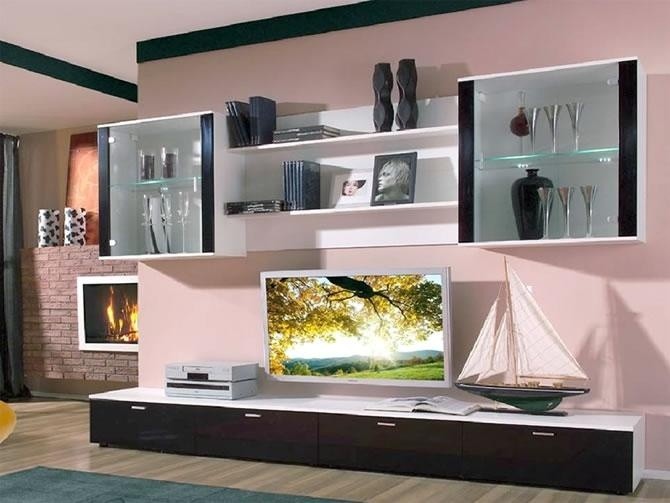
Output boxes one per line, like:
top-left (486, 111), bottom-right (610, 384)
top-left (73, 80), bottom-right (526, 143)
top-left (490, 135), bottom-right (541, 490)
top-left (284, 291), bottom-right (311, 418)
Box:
top-left (95, 109), bottom-right (247, 263)
top-left (220, 93), bottom-right (459, 246)
top-left (457, 56), bottom-right (648, 246)
top-left (90, 384), bottom-right (648, 498)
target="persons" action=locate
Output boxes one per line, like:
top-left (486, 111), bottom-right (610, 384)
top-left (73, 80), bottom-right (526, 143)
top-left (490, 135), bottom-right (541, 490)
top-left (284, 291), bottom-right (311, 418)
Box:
top-left (341, 180), bottom-right (367, 197)
top-left (376, 157), bottom-right (410, 200)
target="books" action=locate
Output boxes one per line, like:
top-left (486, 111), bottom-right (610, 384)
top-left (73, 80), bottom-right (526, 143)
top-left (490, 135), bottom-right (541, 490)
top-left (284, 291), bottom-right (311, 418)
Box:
top-left (363, 394), bottom-right (482, 416)
top-left (282, 159), bottom-right (321, 211)
top-left (225, 198), bottom-right (284, 215)
top-left (223, 94), bottom-right (341, 147)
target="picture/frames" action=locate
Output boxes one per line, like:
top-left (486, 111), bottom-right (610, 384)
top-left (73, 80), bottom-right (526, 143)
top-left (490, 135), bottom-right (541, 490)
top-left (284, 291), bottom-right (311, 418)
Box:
top-left (76, 275), bottom-right (138, 353)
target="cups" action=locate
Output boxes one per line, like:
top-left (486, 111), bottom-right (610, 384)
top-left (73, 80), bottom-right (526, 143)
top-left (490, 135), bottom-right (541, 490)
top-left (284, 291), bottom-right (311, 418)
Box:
top-left (136, 146), bottom-right (155, 182)
top-left (160, 144), bottom-right (179, 180)
top-left (536, 183), bottom-right (601, 242)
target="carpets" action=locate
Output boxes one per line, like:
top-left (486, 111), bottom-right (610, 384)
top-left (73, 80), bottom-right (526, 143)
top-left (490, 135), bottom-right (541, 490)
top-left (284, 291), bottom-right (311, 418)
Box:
top-left (0, 465), bottom-right (365, 503)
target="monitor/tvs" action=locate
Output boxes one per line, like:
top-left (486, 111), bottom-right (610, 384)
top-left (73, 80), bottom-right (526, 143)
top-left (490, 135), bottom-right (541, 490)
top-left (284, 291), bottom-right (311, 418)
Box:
top-left (259, 265), bottom-right (454, 390)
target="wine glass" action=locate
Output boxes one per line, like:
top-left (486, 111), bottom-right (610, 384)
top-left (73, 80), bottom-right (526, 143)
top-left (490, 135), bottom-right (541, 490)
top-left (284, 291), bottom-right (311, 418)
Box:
top-left (521, 99), bottom-right (587, 156)
top-left (140, 190), bottom-right (191, 254)
